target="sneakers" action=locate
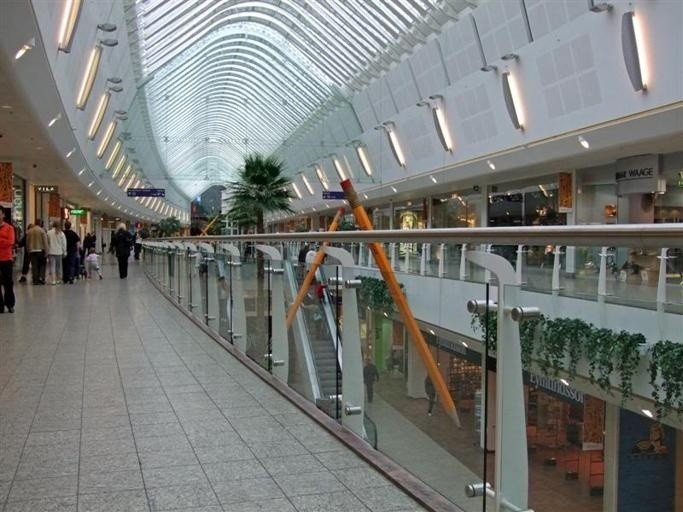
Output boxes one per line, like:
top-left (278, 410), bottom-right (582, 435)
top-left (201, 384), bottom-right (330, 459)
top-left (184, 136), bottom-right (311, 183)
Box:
top-left (18, 273), bottom-right (128, 286)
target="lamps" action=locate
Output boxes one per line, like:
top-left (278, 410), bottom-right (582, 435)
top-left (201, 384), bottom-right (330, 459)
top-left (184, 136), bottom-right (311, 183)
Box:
top-left (650, 176), bottom-right (667, 204)
top-left (587, 0), bottom-right (613, 14)
top-left (101, 23), bottom-right (146, 181)
top-left (293, 53), bottom-right (518, 176)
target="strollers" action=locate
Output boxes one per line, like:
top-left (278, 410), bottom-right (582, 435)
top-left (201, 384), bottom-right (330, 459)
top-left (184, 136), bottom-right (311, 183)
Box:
top-left (77, 266), bottom-right (88, 279)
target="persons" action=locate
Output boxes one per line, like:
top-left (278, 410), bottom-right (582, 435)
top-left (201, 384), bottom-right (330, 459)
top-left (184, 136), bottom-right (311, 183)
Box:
top-left (304, 248), bottom-right (318, 285)
top-left (134, 237), bottom-right (142, 260)
top-left (315, 281), bottom-right (327, 304)
top-left (109, 222), bottom-right (133, 281)
top-left (0, 206), bottom-right (15, 313)
top-left (363, 358), bottom-right (379, 403)
top-left (423, 374), bottom-right (435, 416)
top-left (619, 251), bottom-right (640, 279)
top-left (299, 243), bottom-right (309, 267)
top-left (15, 218), bottom-right (102, 285)
top-left (131, 232), bottom-right (137, 243)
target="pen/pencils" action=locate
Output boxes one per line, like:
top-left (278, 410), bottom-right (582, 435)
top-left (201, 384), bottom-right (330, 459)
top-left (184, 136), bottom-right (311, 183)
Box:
top-left (200, 216), bottom-right (218, 236)
top-left (286, 208), bottom-right (344, 329)
top-left (341, 179), bottom-right (463, 430)
